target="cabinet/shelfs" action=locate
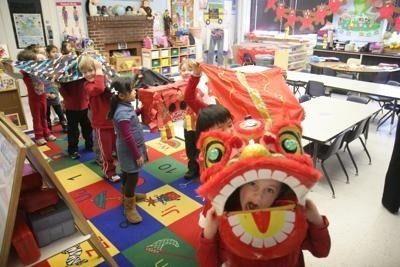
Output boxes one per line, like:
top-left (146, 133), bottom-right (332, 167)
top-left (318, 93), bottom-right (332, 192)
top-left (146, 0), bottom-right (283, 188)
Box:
top-left (232, 37), bottom-right (310, 73)
top-left (310, 48), bottom-right (400, 85)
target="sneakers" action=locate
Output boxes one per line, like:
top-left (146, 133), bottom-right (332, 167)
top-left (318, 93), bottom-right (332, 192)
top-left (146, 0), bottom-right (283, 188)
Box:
top-left (46, 134), bottom-right (56, 141)
top-left (62, 121), bottom-right (67, 126)
top-left (34, 138), bottom-right (47, 145)
top-left (88, 146), bottom-right (96, 152)
top-left (184, 167), bottom-right (198, 179)
top-left (70, 151), bottom-right (81, 159)
top-left (109, 175), bottom-right (121, 182)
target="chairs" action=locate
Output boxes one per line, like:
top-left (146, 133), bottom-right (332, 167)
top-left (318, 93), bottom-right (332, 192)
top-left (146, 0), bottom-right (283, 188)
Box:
top-left (366, 80), bottom-right (400, 121)
top-left (299, 95), bottom-right (311, 103)
top-left (329, 73), bottom-right (355, 94)
top-left (330, 116), bottom-right (372, 176)
top-left (303, 133), bottom-right (350, 198)
top-left (306, 80), bottom-right (326, 98)
top-left (344, 97), bottom-right (368, 152)
top-left (376, 102), bottom-right (400, 135)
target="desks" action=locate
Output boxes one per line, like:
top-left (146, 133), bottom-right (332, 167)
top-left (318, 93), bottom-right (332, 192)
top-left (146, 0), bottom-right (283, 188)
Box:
top-left (300, 95), bottom-right (381, 169)
top-left (232, 64), bottom-right (400, 124)
top-left (313, 63), bottom-right (400, 80)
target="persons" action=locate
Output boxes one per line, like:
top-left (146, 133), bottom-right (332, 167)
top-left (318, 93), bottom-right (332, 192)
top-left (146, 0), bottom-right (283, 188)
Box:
top-left (3, 50), bottom-right (57, 146)
top-left (56, 81), bottom-right (94, 159)
top-left (196, 105), bottom-right (235, 138)
top-left (180, 59), bottom-right (205, 180)
top-left (62, 42), bottom-right (72, 54)
top-left (186, 62), bottom-right (286, 110)
top-left (111, 77), bottom-right (148, 225)
top-left (43, 45), bottom-right (67, 131)
top-left (196, 158), bottom-right (330, 267)
top-left (79, 55), bottom-right (122, 182)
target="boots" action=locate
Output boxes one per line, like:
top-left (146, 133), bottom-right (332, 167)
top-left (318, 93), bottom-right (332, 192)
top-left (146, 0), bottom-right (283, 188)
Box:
top-left (123, 195), bottom-right (142, 223)
top-left (135, 192), bottom-right (146, 201)
